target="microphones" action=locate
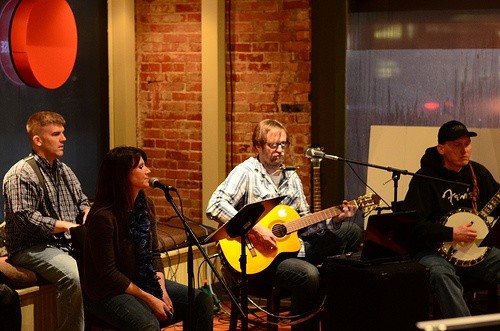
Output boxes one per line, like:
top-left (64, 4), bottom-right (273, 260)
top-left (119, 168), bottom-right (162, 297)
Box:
top-left (305, 148), bottom-right (340, 160)
top-left (149, 177), bottom-right (177, 191)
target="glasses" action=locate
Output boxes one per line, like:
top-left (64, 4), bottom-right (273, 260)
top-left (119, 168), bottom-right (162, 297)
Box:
top-left (267, 142), bottom-right (290, 149)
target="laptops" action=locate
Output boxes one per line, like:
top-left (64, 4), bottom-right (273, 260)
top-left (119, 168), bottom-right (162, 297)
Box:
top-left (333, 210), bottom-right (417, 264)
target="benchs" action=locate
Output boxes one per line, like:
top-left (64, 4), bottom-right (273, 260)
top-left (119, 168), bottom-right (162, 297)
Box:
top-left (0, 216), bottom-right (217, 331)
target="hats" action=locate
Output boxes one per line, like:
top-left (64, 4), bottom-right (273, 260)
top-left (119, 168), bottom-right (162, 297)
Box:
top-left (438, 120), bottom-right (477, 141)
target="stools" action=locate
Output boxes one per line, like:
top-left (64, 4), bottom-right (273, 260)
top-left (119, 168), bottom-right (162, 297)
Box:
top-left (229, 283), bottom-right (292, 331)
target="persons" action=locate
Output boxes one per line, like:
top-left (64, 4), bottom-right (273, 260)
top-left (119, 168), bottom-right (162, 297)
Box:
top-left (403, 120), bottom-right (500, 318)
top-left (2, 111), bottom-right (91, 331)
top-left (206, 118), bottom-right (363, 331)
top-left (83, 147), bottom-right (213, 331)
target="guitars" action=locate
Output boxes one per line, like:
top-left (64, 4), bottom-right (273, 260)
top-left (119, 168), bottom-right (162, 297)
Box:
top-left (437, 188), bottom-right (500, 267)
top-left (216, 193), bottom-right (382, 276)
